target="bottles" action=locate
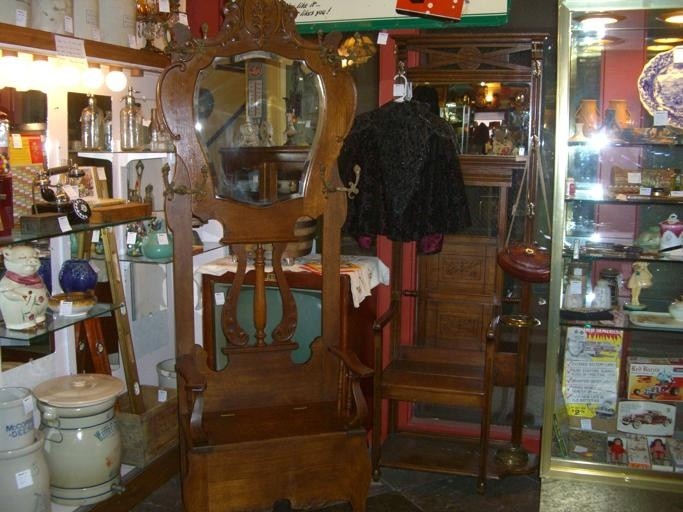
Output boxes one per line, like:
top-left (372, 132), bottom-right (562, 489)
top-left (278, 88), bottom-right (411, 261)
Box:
top-left (591, 278), bottom-right (612, 310)
top-left (102, 111), bottom-right (112, 151)
top-left (674, 171), bottom-right (681, 191)
top-left (147, 108), bottom-right (161, 152)
top-left (566, 176), bottom-right (576, 198)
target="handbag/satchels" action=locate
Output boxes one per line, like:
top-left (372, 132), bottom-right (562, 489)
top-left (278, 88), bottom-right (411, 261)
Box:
top-left (497, 247), bottom-right (550, 282)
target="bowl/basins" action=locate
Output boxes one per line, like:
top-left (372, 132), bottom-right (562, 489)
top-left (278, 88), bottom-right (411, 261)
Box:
top-left (48, 292), bottom-right (98, 316)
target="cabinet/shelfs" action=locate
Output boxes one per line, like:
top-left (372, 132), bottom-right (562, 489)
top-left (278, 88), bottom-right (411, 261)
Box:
top-left (537, 4), bottom-right (682, 512)
top-left (75, 144), bottom-right (229, 320)
top-left (2, 193), bottom-right (189, 508)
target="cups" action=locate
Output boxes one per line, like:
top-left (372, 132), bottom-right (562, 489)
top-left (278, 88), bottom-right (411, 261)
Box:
top-left (574, 99), bottom-right (602, 135)
top-left (606, 99), bottom-right (631, 129)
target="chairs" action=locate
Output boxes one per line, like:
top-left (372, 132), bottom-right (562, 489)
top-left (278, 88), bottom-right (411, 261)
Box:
top-left (149, 1), bottom-right (383, 508)
top-left (368, 234), bottom-right (524, 493)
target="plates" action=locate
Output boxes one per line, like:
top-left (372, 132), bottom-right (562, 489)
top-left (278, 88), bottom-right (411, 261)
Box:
top-left (627, 309), bottom-right (683, 328)
top-left (637, 44), bottom-right (682, 129)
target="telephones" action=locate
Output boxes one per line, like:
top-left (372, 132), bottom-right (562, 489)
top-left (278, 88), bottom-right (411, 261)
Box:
top-left (31, 158), bottom-right (91, 223)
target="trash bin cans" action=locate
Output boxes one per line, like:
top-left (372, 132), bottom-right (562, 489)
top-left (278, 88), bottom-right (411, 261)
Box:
top-left (156, 357), bottom-right (177, 389)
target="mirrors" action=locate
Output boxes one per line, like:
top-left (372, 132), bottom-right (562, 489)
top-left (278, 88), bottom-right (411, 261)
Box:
top-left (192, 50), bottom-right (328, 206)
top-left (413, 82), bottom-right (531, 159)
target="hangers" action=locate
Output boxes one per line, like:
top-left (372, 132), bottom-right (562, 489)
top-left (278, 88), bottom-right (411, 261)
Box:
top-left (383, 75), bottom-right (415, 105)
